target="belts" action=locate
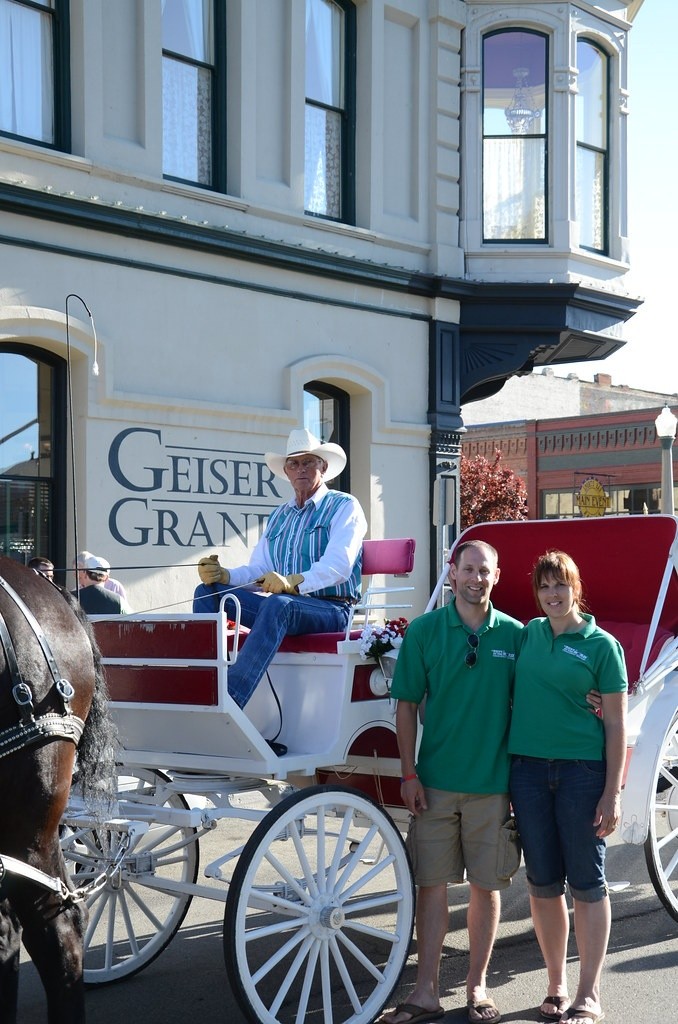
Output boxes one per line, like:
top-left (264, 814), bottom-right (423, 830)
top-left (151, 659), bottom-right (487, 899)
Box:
top-left (314, 595), bottom-right (350, 603)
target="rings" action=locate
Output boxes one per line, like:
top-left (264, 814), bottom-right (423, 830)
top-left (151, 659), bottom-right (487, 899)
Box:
top-left (613, 825), bottom-right (618, 828)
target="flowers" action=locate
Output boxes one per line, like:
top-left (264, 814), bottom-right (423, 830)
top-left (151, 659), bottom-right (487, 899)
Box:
top-left (357, 617), bottom-right (409, 662)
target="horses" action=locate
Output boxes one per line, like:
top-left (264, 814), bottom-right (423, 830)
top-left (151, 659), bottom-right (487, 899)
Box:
top-left (0, 556), bottom-right (117, 1024)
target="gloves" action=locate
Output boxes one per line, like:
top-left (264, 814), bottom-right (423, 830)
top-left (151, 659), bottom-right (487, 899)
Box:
top-left (253, 571), bottom-right (304, 596)
top-left (198, 555), bottom-right (229, 585)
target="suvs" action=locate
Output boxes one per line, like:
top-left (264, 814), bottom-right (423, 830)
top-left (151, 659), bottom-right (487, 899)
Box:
top-left (0, 513), bottom-right (678, 1024)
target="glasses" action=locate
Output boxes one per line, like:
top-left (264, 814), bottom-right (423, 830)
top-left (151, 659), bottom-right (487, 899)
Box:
top-left (285, 459), bottom-right (322, 470)
top-left (465, 633), bottom-right (479, 668)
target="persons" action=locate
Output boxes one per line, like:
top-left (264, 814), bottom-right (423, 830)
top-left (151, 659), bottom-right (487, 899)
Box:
top-left (70, 557), bottom-right (131, 615)
top-left (193, 429), bottom-right (368, 710)
top-left (72, 551), bottom-right (126, 599)
top-left (28, 557), bottom-right (54, 582)
top-left (508, 551), bottom-right (628, 1024)
top-left (379, 540), bottom-right (602, 1024)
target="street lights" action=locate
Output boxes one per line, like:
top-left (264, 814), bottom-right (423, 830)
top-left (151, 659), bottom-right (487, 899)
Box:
top-left (654, 402), bottom-right (678, 517)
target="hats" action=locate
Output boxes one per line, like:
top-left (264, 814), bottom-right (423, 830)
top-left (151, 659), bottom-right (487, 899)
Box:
top-left (83, 557), bottom-right (110, 575)
top-left (265, 429), bottom-right (347, 482)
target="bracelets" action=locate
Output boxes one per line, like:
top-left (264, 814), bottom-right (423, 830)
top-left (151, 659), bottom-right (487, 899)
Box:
top-left (400, 774), bottom-right (418, 783)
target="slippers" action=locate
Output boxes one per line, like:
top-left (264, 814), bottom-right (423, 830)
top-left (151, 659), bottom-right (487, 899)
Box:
top-left (379, 1003), bottom-right (444, 1024)
top-left (467, 996), bottom-right (501, 1024)
top-left (540, 994), bottom-right (572, 1019)
top-left (565, 1007), bottom-right (605, 1024)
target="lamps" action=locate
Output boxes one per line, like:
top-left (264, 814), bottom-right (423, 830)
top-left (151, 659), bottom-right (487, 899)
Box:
top-left (503, 66), bottom-right (543, 134)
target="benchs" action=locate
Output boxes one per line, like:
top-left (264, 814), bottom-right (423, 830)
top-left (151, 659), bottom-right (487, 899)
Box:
top-left (424, 514), bottom-right (678, 699)
top-left (229, 538), bottom-right (415, 655)
